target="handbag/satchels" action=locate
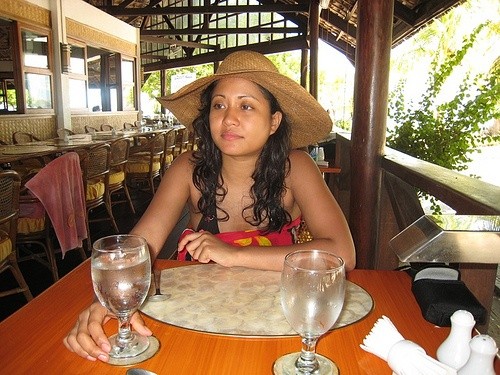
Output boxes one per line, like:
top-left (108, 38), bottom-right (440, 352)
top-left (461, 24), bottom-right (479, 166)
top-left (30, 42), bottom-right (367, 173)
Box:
top-left (394, 261), bottom-right (487, 328)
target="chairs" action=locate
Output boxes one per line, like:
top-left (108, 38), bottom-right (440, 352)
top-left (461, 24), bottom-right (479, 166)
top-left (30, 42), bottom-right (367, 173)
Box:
top-left (0, 120), bottom-right (191, 302)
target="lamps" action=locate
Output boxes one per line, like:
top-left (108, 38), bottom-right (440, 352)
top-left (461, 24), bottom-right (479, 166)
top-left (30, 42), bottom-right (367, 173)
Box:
top-left (61, 42), bottom-right (72, 74)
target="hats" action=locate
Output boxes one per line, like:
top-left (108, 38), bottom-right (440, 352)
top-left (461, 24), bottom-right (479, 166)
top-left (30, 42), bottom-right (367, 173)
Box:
top-left (154, 49), bottom-right (334, 151)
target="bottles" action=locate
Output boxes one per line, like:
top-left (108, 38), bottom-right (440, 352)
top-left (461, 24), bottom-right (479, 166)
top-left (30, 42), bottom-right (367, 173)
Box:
top-left (436, 310), bottom-right (476, 371)
top-left (457, 335), bottom-right (498, 375)
top-left (152, 119), bottom-right (168, 131)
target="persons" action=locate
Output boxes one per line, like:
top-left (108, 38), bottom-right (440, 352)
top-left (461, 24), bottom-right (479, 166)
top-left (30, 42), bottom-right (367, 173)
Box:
top-left (63, 50), bottom-right (356, 362)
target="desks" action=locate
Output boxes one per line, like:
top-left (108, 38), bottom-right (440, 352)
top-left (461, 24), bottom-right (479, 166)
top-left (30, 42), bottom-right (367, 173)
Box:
top-left (101, 129), bottom-right (158, 134)
top-left (11, 138), bottom-right (109, 149)
top-left (67, 131), bottom-right (131, 140)
top-left (0, 145), bottom-right (75, 165)
top-left (0, 254), bottom-right (500, 375)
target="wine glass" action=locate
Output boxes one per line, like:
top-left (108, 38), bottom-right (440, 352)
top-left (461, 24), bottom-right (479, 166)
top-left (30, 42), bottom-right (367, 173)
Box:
top-left (279, 250), bottom-right (346, 375)
top-left (91, 235), bottom-right (152, 358)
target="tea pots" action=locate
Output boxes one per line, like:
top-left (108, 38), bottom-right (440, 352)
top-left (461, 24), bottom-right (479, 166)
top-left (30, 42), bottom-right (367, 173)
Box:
top-left (318, 147), bottom-right (324, 160)
